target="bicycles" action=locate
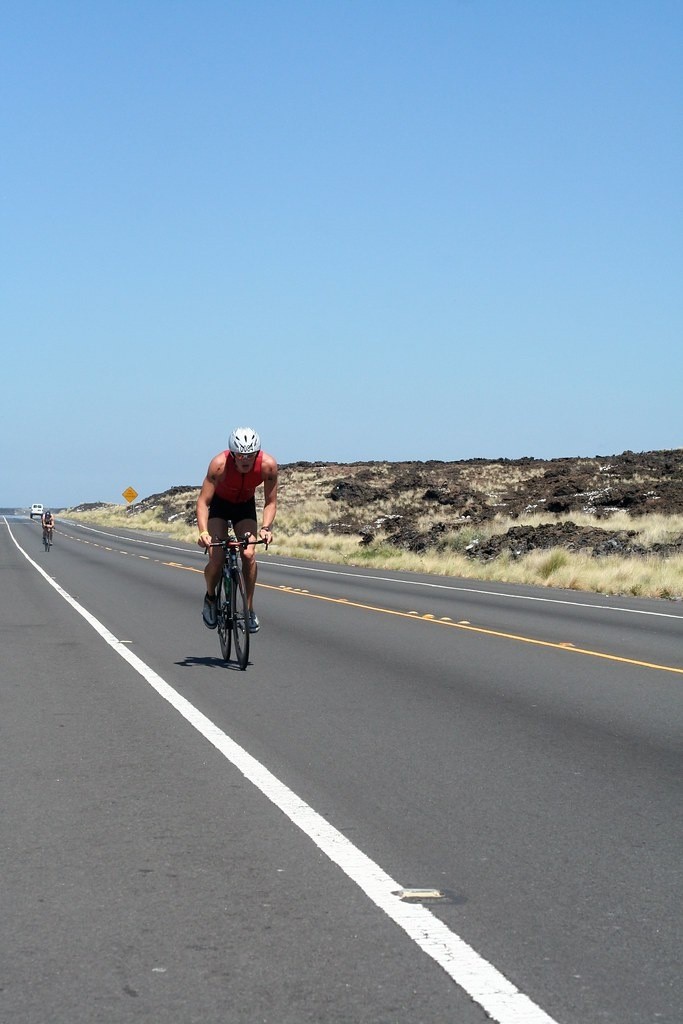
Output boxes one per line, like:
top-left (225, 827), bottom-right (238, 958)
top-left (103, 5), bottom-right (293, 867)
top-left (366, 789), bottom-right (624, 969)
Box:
top-left (204, 532), bottom-right (268, 671)
top-left (42, 525), bottom-right (55, 552)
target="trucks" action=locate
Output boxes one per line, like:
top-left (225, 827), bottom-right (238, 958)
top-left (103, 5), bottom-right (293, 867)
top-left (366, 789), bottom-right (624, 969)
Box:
top-left (29, 504), bottom-right (44, 519)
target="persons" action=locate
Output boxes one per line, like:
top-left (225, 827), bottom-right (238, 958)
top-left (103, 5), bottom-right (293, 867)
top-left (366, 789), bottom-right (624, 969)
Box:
top-left (41, 511), bottom-right (55, 546)
top-left (196, 427), bottom-right (277, 632)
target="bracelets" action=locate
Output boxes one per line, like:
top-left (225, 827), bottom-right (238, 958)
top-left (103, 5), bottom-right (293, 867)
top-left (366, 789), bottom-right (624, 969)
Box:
top-left (261, 526), bottom-right (270, 532)
top-left (200, 531), bottom-right (209, 536)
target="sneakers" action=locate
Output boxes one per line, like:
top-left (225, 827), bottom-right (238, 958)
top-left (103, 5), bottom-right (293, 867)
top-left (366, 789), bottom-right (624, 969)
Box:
top-left (248, 612), bottom-right (260, 633)
top-left (203, 591), bottom-right (217, 629)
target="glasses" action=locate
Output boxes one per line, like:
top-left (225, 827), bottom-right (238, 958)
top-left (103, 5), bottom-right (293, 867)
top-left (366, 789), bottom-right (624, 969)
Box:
top-left (234, 453), bottom-right (257, 460)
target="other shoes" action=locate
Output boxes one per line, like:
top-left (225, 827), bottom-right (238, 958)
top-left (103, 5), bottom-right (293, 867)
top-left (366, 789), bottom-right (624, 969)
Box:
top-left (49, 542), bottom-right (53, 545)
top-left (43, 538), bottom-right (46, 544)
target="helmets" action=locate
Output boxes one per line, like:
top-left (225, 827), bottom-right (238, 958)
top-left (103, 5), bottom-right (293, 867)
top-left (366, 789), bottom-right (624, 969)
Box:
top-left (229, 427), bottom-right (261, 453)
top-left (46, 511), bottom-right (50, 516)
